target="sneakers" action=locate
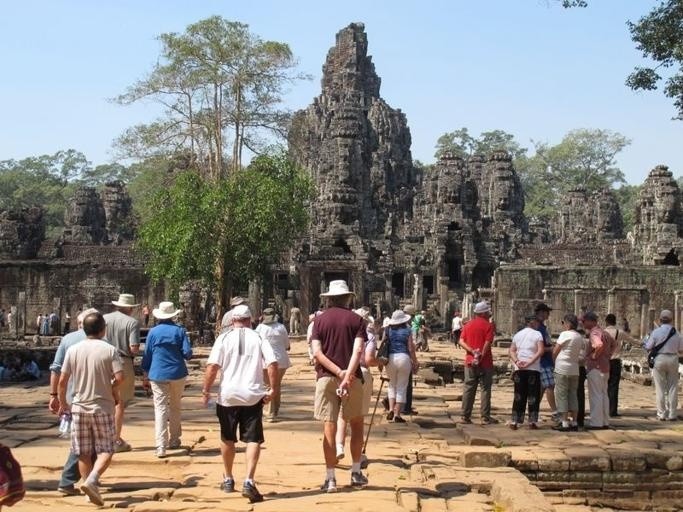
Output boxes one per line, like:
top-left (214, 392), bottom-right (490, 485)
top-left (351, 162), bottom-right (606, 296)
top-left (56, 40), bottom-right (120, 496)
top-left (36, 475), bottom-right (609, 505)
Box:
top-left (242, 480), bottom-right (264, 502)
top-left (86, 493), bottom-right (91, 502)
top-left (351, 472), bottom-right (368, 484)
top-left (157, 446), bottom-right (166, 457)
top-left (460, 416), bottom-right (473, 424)
top-left (220, 479), bottom-right (234, 492)
top-left (264, 416), bottom-right (274, 422)
top-left (481, 415), bottom-right (498, 425)
top-left (361, 454), bottom-right (368, 469)
top-left (336, 444), bottom-right (344, 459)
top-left (58, 485), bottom-right (81, 495)
top-left (112, 439), bottom-right (132, 453)
top-left (669, 418), bottom-right (676, 420)
top-left (277, 411), bottom-right (280, 416)
top-left (320, 478), bottom-right (337, 492)
top-left (170, 439), bottom-right (181, 448)
top-left (81, 480), bottom-right (105, 506)
top-left (659, 417), bottom-right (665, 421)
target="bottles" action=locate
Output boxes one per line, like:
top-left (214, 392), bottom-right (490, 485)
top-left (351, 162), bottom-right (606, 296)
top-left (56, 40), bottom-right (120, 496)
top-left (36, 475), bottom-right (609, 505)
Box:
top-left (58, 408), bottom-right (70, 436)
top-left (207, 400), bottom-right (216, 410)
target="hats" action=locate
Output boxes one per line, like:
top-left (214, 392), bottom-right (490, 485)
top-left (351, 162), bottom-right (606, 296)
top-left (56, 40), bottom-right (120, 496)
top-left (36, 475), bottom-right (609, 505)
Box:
top-left (403, 305), bottom-right (415, 316)
top-left (355, 308), bottom-right (372, 323)
top-left (231, 296), bottom-right (249, 306)
top-left (259, 308), bottom-right (280, 323)
top-left (387, 310), bottom-right (411, 325)
top-left (153, 302), bottom-right (182, 319)
top-left (473, 302), bottom-right (491, 313)
top-left (525, 315), bottom-right (537, 323)
top-left (606, 314), bottom-right (616, 325)
top-left (319, 280), bottom-right (357, 297)
top-left (659, 310), bottom-right (672, 320)
top-left (583, 311), bottom-right (598, 321)
top-left (232, 305), bottom-right (252, 318)
top-left (77, 308), bottom-right (99, 323)
top-left (576, 320), bottom-right (585, 335)
top-left (112, 293), bottom-right (142, 307)
top-left (533, 303), bottom-right (552, 311)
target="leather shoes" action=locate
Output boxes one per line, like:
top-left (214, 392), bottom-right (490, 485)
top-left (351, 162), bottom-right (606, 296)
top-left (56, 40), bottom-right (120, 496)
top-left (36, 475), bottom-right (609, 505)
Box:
top-left (584, 424), bottom-right (600, 429)
top-left (381, 397), bottom-right (389, 409)
top-left (507, 423), bottom-right (517, 430)
top-left (552, 423), bottom-right (569, 431)
top-left (611, 412), bottom-right (621, 417)
top-left (387, 411), bottom-right (394, 421)
top-left (395, 416), bottom-right (406, 422)
top-left (405, 410), bottom-right (418, 415)
top-left (602, 425), bottom-right (608, 429)
top-left (528, 423), bottom-right (539, 429)
top-left (569, 424), bottom-right (577, 432)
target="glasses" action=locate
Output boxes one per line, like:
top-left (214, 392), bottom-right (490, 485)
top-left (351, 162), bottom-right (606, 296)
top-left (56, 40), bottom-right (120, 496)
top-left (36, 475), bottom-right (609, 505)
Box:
top-left (561, 320), bottom-right (566, 325)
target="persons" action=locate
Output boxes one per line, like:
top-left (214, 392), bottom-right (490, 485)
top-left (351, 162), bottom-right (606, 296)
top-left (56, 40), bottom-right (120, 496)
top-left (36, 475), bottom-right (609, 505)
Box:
top-left (202, 305), bottom-right (280, 502)
top-left (381, 304), bottom-right (419, 415)
top-left (535, 304), bottom-right (559, 421)
top-left (289, 304), bottom-right (301, 335)
top-left (385, 309), bottom-right (419, 423)
top-left (452, 311), bottom-right (464, 349)
top-left (576, 323), bottom-right (588, 426)
top-left (380, 315), bottom-right (391, 339)
top-left (142, 302), bottom-right (193, 458)
top-left (307, 314), bottom-right (315, 366)
top-left (551, 315), bottom-right (586, 432)
top-left (58, 313), bottom-right (124, 506)
top-left (507, 316), bottom-right (544, 431)
top-left (220, 297), bottom-right (252, 334)
top-left (101, 294), bottom-right (140, 452)
top-left (362, 306), bottom-right (374, 326)
top-left (418, 311), bottom-right (433, 351)
top-left (0, 444), bottom-right (25, 512)
top-left (602, 314), bottom-right (645, 417)
top-left (143, 304), bottom-right (149, 328)
top-left (0, 308), bottom-right (82, 336)
top-left (49, 307), bottom-right (99, 495)
top-left (458, 303), bottom-right (499, 425)
top-left (582, 313), bottom-right (619, 431)
top-left (255, 308), bottom-right (293, 422)
top-left (336, 309), bottom-right (388, 469)
top-left (645, 310), bottom-right (683, 422)
top-left (311, 280), bottom-right (367, 492)
